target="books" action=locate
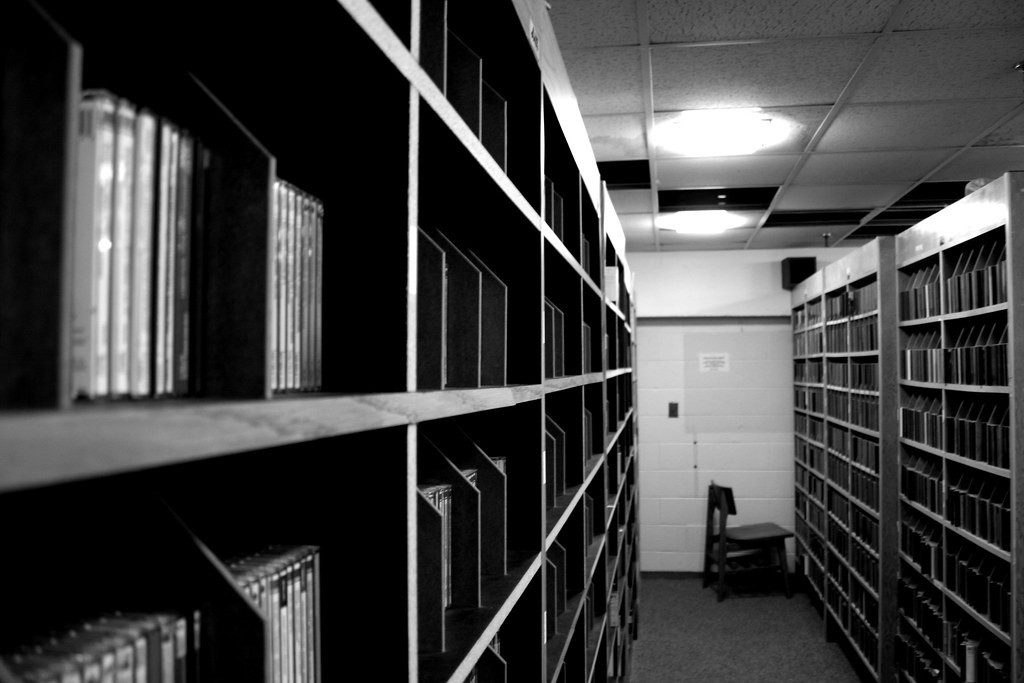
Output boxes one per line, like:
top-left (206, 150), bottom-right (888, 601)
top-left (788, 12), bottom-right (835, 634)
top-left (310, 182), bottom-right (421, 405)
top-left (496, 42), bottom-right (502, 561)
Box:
top-left (48, 88), bottom-right (329, 396)
top-left (418, 457), bottom-right (508, 612)
top-left (1, 541), bottom-right (322, 683)
top-left (605, 265), bottom-right (619, 304)
top-left (791, 227), bottom-right (1009, 683)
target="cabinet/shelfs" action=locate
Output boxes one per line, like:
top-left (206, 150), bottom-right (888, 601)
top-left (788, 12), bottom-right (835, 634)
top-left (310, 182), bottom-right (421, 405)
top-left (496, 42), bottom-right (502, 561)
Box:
top-left (791, 270), bottom-right (825, 635)
top-left (823, 236), bottom-right (897, 683)
top-left (0, 0), bottom-right (610, 683)
top-left (601, 180), bottom-right (641, 683)
top-left (894, 171), bottom-right (1024, 683)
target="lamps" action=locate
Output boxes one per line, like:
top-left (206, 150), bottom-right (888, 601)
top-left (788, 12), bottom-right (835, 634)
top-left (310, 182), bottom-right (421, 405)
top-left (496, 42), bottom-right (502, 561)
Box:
top-left (655, 209), bottom-right (748, 236)
top-left (647, 104), bottom-right (794, 160)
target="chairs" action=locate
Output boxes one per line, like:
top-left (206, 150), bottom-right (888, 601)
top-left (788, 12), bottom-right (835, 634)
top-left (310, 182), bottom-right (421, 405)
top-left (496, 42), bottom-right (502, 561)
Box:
top-left (702, 479), bottom-right (794, 604)
